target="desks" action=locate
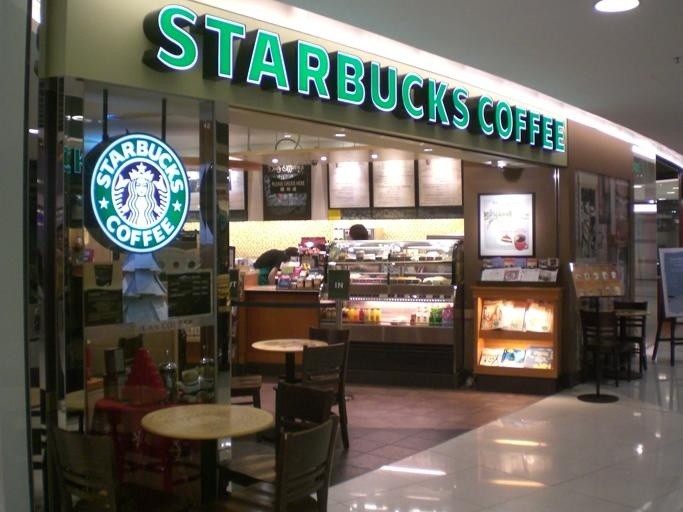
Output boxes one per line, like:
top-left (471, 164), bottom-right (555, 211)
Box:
top-left (142, 403), bottom-right (274, 512)
top-left (251, 339), bottom-right (328, 380)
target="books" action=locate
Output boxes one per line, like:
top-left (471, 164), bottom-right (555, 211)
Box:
top-left (479, 257), bottom-right (559, 369)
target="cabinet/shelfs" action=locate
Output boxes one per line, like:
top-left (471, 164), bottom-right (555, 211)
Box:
top-left (470, 286), bottom-right (565, 391)
top-left (318, 237), bottom-right (465, 391)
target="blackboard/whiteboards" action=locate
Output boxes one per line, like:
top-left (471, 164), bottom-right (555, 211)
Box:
top-left (658, 248), bottom-right (683, 318)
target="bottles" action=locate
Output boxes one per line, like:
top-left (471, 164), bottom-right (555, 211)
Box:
top-left (340, 307), bottom-right (380, 323)
top-left (415, 307), bottom-right (442, 326)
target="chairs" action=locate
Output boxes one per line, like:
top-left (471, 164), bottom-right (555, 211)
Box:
top-left (230, 375), bottom-right (264, 410)
top-left (218, 414), bottom-right (338, 512)
top-left (308, 327), bottom-right (348, 346)
top-left (580, 300), bottom-right (649, 386)
top-left (301, 341), bottom-right (349, 450)
top-left (47, 424), bottom-right (191, 512)
top-left (272, 381), bottom-right (333, 450)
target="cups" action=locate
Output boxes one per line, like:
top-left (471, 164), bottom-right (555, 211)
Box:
top-left (514, 234), bottom-right (528, 250)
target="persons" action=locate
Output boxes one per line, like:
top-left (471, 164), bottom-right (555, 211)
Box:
top-left (252, 246), bottom-right (299, 285)
top-left (349, 224), bottom-right (368, 240)
